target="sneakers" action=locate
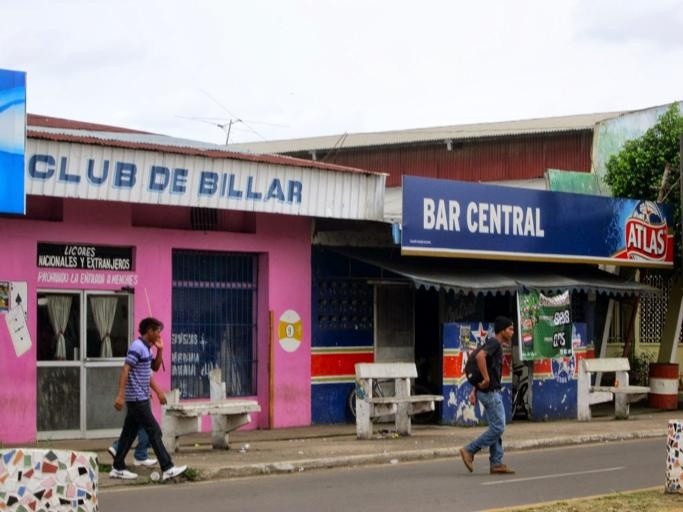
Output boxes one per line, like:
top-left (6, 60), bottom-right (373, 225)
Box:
top-left (108, 446), bottom-right (188, 481)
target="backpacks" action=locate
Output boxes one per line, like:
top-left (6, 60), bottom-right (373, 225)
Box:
top-left (464, 338), bottom-right (488, 386)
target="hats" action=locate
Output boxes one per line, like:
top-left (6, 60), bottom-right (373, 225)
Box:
top-left (493, 317), bottom-right (513, 335)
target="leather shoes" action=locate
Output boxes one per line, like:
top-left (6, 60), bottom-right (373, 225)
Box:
top-left (459, 448), bottom-right (476, 472)
top-left (489, 462), bottom-right (515, 474)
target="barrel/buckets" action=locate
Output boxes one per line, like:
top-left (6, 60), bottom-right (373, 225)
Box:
top-left (649, 363), bottom-right (680, 410)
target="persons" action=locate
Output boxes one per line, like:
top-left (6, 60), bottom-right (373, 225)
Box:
top-left (107, 367), bottom-right (169, 467)
top-left (456, 316), bottom-right (517, 475)
top-left (106, 315), bottom-right (189, 482)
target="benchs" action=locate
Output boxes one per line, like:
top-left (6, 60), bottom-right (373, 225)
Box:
top-left (353, 360), bottom-right (446, 441)
top-left (575, 355), bottom-right (653, 424)
top-left (159, 367), bottom-right (263, 454)
